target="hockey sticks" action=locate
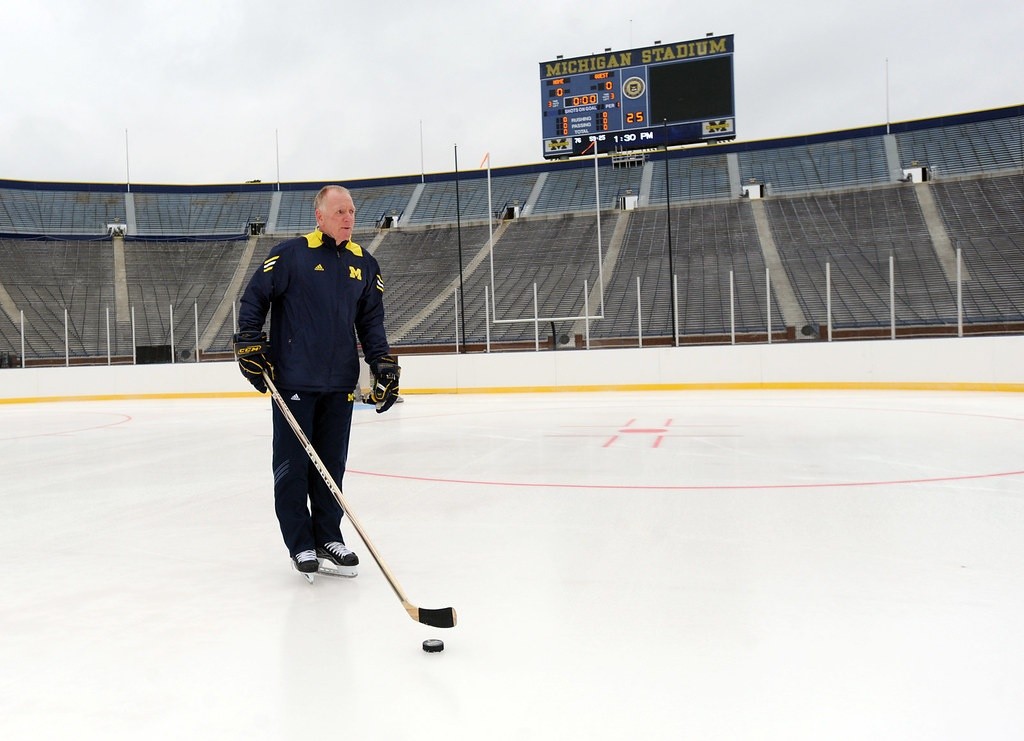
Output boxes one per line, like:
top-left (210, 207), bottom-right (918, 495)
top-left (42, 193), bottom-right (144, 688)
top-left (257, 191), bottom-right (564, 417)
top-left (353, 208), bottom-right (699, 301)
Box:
top-left (259, 367), bottom-right (458, 629)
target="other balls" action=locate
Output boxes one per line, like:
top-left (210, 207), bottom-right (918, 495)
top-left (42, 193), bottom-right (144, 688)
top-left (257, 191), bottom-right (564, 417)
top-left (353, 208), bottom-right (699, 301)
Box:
top-left (422, 638), bottom-right (444, 653)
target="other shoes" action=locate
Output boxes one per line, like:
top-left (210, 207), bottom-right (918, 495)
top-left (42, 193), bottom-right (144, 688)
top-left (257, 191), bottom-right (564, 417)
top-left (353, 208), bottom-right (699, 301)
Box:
top-left (292, 549), bottom-right (320, 583)
top-left (317, 538), bottom-right (359, 577)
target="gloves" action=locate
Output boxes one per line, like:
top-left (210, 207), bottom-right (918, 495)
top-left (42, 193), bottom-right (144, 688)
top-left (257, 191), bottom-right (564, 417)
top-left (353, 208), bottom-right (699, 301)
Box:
top-left (362, 356), bottom-right (401, 413)
top-left (233, 331), bottom-right (272, 393)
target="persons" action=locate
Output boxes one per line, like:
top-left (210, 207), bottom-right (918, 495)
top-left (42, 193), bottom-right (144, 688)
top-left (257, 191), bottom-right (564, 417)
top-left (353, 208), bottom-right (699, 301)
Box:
top-left (232, 185), bottom-right (401, 583)
